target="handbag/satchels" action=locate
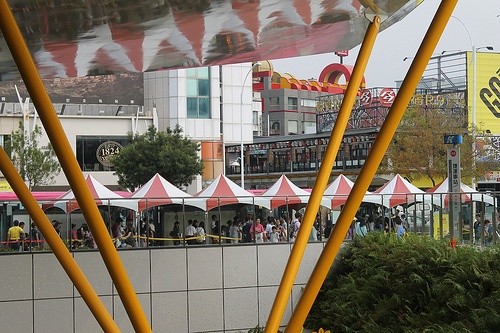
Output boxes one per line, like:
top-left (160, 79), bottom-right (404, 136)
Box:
top-left (169, 230), bottom-right (177, 236)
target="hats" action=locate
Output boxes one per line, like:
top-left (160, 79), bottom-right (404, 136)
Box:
top-left (115, 217), bottom-right (124, 222)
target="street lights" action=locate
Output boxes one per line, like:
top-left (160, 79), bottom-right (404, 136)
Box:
top-left (240, 62), bottom-right (261, 189)
top-left (472, 44), bottom-right (494, 242)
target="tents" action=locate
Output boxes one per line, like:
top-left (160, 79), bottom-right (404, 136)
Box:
top-left (426, 175), bottom-right (498, 228)
top-left (191, 174), bottom-right (271, 244)
top-left (316, 174), bottom-right (391, 234)
top-left (124, 172), bottom-right (208, 246)
top-left (374, 175), bottom-right (444, 236)
top-left (258, 174), bottom-right (333, 225)
top-left (40, 173), bottom-right (139, 251)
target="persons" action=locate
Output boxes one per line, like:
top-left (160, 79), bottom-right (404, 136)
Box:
top-left (183, 210), bottom-right (411, 244)
top-left (6, 220), bottom-right (25, 252)
top-left (171, 221), bottom-right (182, 245)
top-left (18, 218), bottom-right (155, 248)
top-left (474, 212), bottom-right (495, 247)
